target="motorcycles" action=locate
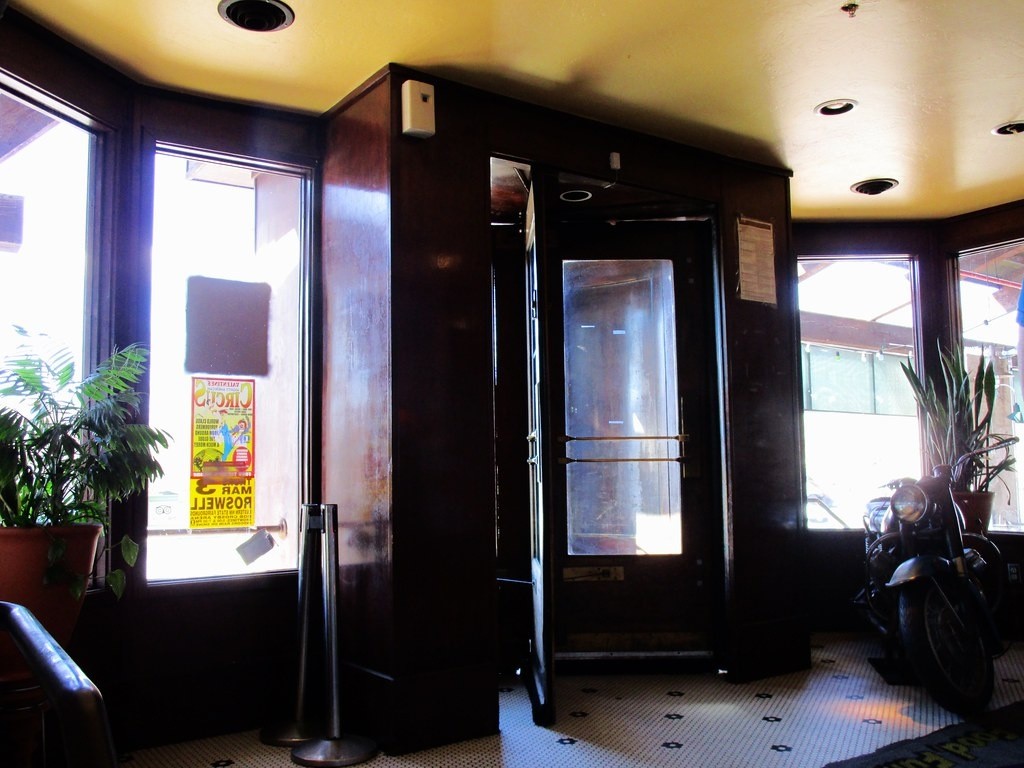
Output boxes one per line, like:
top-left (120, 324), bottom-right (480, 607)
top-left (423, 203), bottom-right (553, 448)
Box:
top-left (850, 434), bottom-right (1018, 716)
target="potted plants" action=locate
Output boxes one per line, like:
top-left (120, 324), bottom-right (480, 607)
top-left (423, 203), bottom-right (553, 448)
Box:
top-left (0, 326), bottom-right (175, 683)
top-left (899, 337), bottom-right (1019, 534)
